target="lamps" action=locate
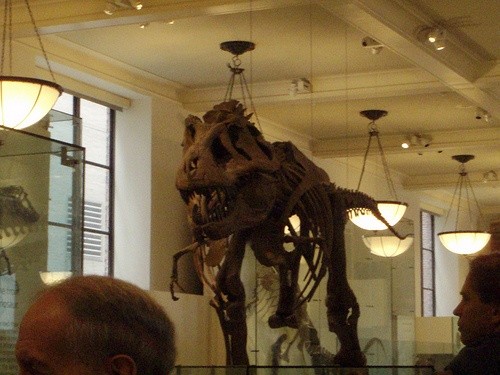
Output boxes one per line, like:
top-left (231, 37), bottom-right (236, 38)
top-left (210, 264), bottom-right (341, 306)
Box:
top-left (0, 0), bottom-right (63, 133)
top-left (346, 107), bottom-right (410, 235)
top-left (427, 28), bottom-right (448, 51)
top-left (436, 155), bottom-right (492, 255)
top-left (362, 235), bottom-right (414, 258)
top-left (104, 0), bottom-right (144, 17)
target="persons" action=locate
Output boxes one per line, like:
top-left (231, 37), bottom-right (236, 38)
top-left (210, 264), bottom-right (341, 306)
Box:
top-left (437, 252), bottom-right (500, 375)
top-left (16, 275), bottom-right (176, 375)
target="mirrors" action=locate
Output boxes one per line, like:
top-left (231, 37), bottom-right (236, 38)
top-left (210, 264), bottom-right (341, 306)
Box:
top-left (255, 147), bottom-right (500, 367)
